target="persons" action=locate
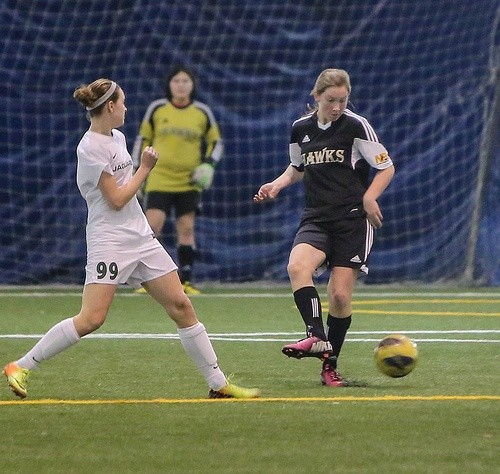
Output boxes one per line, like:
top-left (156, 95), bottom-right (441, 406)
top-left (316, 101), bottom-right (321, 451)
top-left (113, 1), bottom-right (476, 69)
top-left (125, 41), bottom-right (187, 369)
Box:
top-left (0, 78), bottom-right (262, 399)
top-left (254, 68), bottom-right (395, 387)
top-left (132, 68), bottom-right (224, 296)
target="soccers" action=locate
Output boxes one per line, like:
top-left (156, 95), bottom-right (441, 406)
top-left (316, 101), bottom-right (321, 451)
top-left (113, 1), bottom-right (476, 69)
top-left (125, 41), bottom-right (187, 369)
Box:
top-left (374, 334), bottom-right (418, 378)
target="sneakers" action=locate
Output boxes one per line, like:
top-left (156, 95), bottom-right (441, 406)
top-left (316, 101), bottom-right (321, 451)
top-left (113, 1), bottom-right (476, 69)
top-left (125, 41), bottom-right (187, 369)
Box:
top-left (320, 363), bottom-right (346, 388)
top-left (182, 282), bottom-right (201, 296)
top-left (208, 378), bottom-right (261, 399)
top-left (131, 287), bottom-right (147, 294)
top-left (281, 331), bottom-right (334, 362)
top-left (2, 361), bottom-right (32, 400)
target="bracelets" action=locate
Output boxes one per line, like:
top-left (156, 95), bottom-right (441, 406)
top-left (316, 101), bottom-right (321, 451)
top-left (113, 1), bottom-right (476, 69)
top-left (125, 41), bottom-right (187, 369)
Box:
top-left (206, 156), bottom-right (219, 169)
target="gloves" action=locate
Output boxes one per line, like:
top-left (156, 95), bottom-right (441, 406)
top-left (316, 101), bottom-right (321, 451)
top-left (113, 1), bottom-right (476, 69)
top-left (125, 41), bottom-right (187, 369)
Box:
top-left (132, 168), bottom-right (145, 199)
top-left (191, 163), bottom-right (214, 190)
